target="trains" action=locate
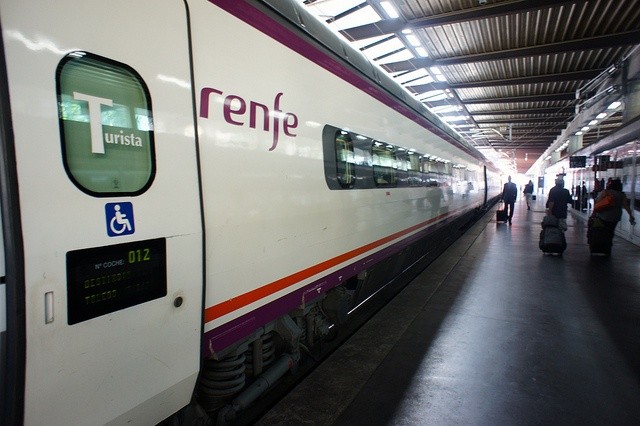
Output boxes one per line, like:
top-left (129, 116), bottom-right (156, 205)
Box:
top-left (1, 1), bottom-right (505, 426)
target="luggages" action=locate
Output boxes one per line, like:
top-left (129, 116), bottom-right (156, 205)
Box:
top-left (539, 214), bottom-right (566, 253)
top-left (587, 214), bottom-right (609, 253)
top-left (497, 202), bottom-right (506, 221)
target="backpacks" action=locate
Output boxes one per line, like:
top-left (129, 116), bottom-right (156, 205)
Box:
top-left (589, 215), bottom-right (604, 236)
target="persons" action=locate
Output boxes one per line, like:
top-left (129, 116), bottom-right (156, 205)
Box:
top-left (524, 180), bottom-right (534, 210)
top-left (546, 176), bottom-right (574, 230)
top-left (501, 176), bottom-right (516, 225)
top-left (574, 178), bottom-right (635, 246)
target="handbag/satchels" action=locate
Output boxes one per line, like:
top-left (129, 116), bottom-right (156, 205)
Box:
top-left (595, 189), bottom-right (615, 213)
top-left (542, 214), bottom-right (559, 228)
